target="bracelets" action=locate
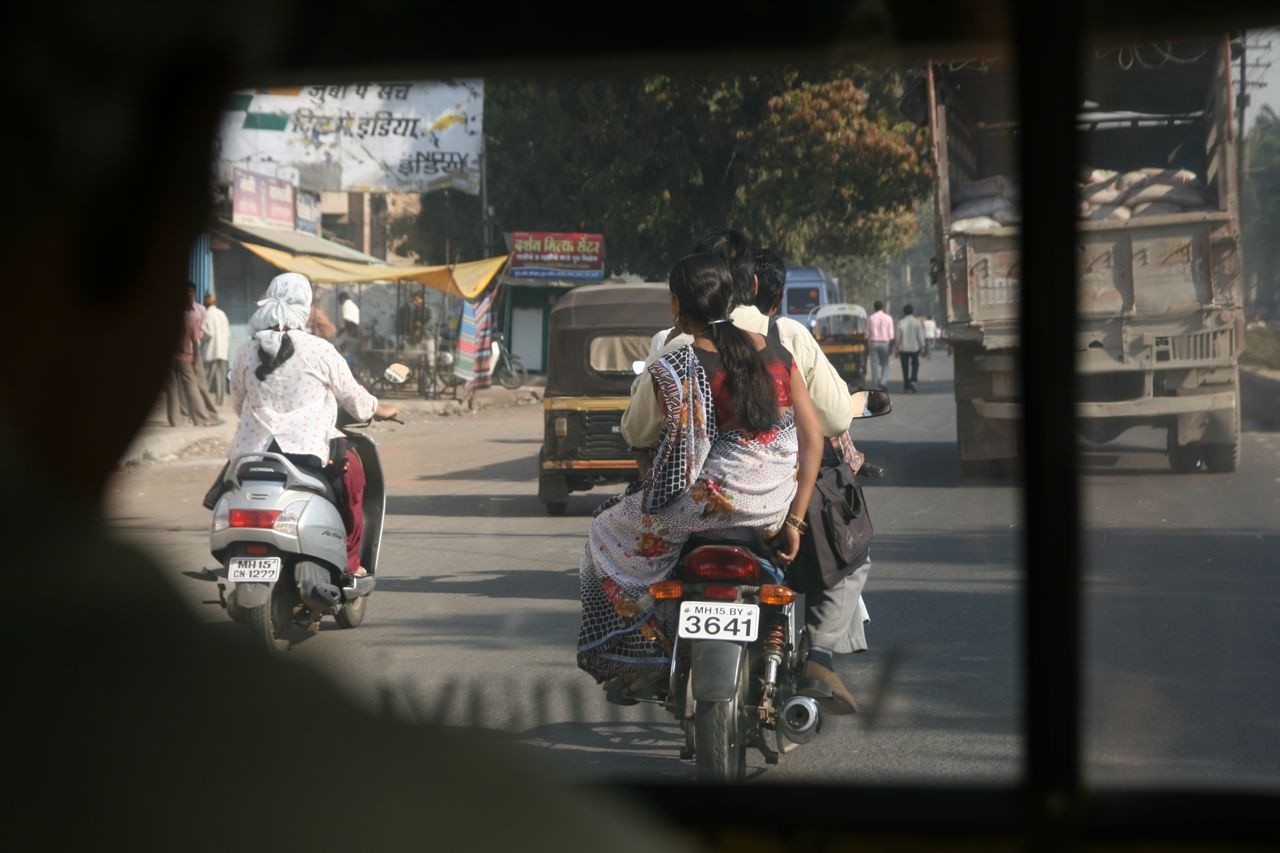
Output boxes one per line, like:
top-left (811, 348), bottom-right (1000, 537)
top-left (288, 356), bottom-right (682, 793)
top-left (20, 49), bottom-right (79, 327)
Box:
top-left (786, 514), bottom-right (808, 535)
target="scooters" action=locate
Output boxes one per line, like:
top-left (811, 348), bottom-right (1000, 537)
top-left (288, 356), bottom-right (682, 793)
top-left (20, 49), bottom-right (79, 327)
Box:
top-left (205, 361), bottom-right (411, 664)
top-left (492, 324), bottom-right (529, 390)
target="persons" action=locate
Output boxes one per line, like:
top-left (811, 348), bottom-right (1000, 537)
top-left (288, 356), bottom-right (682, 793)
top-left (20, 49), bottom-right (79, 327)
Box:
top-left (866, 301), bottom-right (894, 391)
top-left (647, 231), bottom-right (870, 714)
top-left (229, 272), bottom-right (397, 578)
top-left (165, 281), bottom-right (223, 425)
top-left (410, 293), bottom-right (436, 370)
top-left (332, 293), bottom-right (360, 347)
top-left (575, 256), bottom-right (823, 708)
top-left (199, 294), bottom-right (228, 405)
top-left (924, 316), bottom-right (937, 351)
top-left (895, 303), bottom-right (926, 391)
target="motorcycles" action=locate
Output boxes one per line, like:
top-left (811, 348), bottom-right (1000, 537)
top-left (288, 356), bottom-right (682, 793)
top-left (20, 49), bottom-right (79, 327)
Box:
top-left (532, 278), bottom-right (676, 515)
top-left (625, 387), bottom-right (892, 810)
top-left (807, 302), bottom-right (871, 385)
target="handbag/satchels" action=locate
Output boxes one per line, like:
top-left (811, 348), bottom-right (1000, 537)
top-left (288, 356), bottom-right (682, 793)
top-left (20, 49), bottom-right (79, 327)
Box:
top-left (808, 439), bottom-right (873, 589)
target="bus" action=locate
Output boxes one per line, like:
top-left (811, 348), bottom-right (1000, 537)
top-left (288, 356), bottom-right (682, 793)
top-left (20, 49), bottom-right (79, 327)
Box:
top-left (776, 267), bottom-right (844, 344)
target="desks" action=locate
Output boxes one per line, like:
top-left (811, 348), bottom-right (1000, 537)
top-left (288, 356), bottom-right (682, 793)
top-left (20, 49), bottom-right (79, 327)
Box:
top-left (360, 348), bottom-right (428, 396)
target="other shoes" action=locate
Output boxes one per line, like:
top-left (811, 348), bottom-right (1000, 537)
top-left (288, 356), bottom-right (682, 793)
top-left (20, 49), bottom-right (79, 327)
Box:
top-left (903, 382), bottom-right (918, 393)
top-left (622, 668), bottom-right (670, 700)
top-left (801, 661), bottom-right (858, 716)
top-left (355, 566), bottom-right (368, 578)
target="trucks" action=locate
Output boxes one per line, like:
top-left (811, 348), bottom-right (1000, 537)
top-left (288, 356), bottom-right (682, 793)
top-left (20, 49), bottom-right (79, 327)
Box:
top-left (920, 22), bottom-right (1247, 478)
